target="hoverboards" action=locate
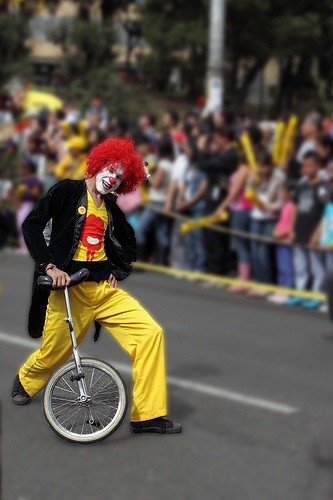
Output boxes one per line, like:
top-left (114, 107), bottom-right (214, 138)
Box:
top-left (36, 268), bottom-right (128, 444)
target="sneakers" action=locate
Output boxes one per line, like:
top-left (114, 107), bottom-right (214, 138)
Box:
top-left (130, 413), bottom-right (183, 434)
top-left (11, 374), bottom-right (31, 406)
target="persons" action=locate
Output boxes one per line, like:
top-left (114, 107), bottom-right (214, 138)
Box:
top-left (10, 139), bottom-right (183, 435)
top-left (0, 84), bottom-right (333, 315)
